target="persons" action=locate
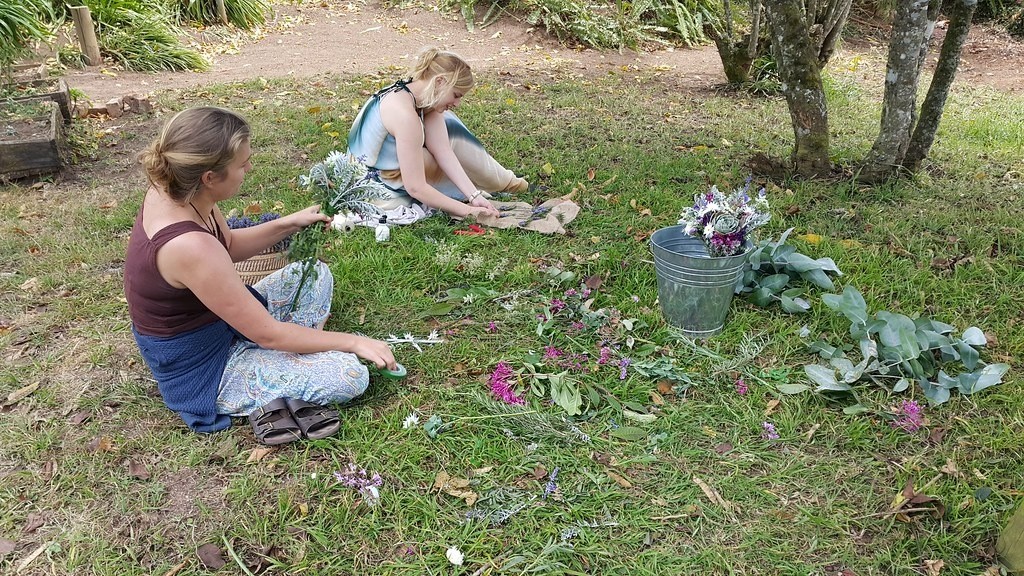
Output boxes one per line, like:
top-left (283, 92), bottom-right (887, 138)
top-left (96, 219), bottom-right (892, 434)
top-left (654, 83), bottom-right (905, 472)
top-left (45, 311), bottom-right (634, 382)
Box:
top-left (349, 47), bottom-right (530, 223)
top-left (124, 106), bottom-right (398, 433)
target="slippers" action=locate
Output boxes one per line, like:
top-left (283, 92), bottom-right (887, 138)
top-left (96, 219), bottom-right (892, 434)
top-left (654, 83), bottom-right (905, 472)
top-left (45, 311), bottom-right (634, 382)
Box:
top-left (286, 399), bottom-right (340, 441)
top-left (249, 398), bottom-right (301, 446)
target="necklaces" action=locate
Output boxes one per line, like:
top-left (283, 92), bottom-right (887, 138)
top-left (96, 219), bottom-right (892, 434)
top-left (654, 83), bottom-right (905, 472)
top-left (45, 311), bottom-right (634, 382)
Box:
top-left (188, 199), bottom-right (219, 237)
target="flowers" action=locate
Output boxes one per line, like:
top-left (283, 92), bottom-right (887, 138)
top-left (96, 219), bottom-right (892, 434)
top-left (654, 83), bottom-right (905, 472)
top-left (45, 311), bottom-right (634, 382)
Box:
top-left (227, 213), bottom-right (292, 254)
top-left (677, 173), bottom-right (772, 257)
top-left (270, 151), bottom-right (391, 317)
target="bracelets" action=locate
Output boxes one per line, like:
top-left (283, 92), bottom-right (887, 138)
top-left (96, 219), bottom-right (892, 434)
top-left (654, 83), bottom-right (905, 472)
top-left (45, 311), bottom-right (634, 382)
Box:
top-left (468, 190), bottom-right (482, 204)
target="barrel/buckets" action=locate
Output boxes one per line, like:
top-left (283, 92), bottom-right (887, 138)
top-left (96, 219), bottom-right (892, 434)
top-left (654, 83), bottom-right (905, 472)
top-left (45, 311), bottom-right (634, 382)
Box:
top-left (650, 226), bottom-right (753, 336)
